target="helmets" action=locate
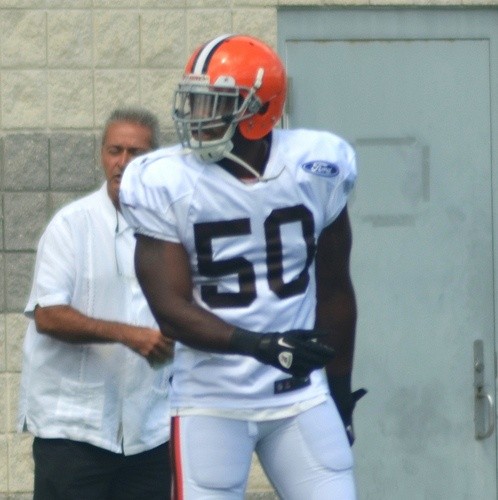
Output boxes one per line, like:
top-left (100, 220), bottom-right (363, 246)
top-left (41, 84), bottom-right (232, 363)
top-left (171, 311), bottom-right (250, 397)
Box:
top-left (181, 32), bottom-right (286, 140)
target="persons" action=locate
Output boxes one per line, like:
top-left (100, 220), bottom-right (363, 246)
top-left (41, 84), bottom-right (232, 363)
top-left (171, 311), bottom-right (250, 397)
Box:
top-left (119, 34), bottom-right (366, 500)
top-left (17, 109), bottom-right (175, 500)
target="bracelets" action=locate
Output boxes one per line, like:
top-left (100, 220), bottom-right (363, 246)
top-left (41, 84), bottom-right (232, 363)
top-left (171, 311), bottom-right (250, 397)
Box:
top-left (330, 374), bottom-right (350, 400)
top-left (230, 327), bottom-right (263, 355)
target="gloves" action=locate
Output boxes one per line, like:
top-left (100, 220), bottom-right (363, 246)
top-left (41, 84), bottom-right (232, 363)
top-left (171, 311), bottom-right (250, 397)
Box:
top-left (228, 326), bottom-right (338, 380)
top-left (328, 370), bottom-right (366, 448)
